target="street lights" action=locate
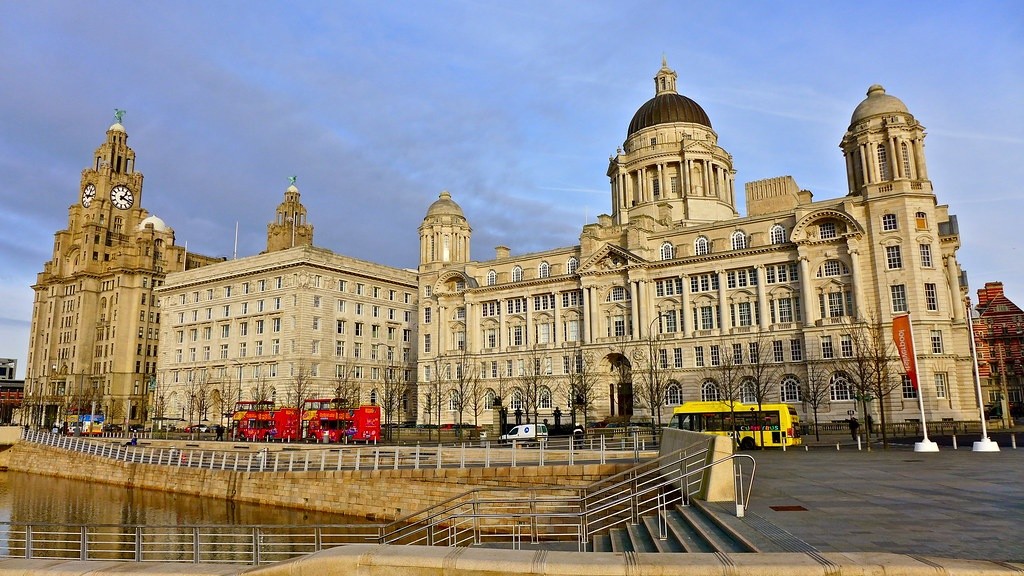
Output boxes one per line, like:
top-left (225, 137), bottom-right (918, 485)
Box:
top-left (229, 359), bottom-right (243, 410)
top-left (649, 313), bottom-right (670, 446)
top-left (371, 342), bottom-right (393, 442)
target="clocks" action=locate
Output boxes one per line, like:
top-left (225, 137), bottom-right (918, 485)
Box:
top-left (109, 184), bottom-right (134, 209)
top-left (82, 184), bottom-right (96, 208)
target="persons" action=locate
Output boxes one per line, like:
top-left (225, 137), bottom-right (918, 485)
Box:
top-left (205, 427), bottom-right (209, 434)
top-left (572, 426), bottom-right (584, 449)
top-left (849, 417), bottom-right (858, 441)
top-left (865, 413), bottom-right (873, 438)
top-left (216, 424), bottom-right (224, 441)
top-left (131, 434), bottom-right (137, 446)
top-left (232, 426), bottom-right (237, 441)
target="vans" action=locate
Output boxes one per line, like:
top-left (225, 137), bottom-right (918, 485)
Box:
top-left (498, 424), bottom-right (548, 450)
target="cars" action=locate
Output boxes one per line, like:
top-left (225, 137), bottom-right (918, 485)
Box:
top-left (379, 422), bottom-right (481, 432)
top-left (103, 420), bottom-right (230, 434)
top-left (586, 421), bottom-right (678, 436)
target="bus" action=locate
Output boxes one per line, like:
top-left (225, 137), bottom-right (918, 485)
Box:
top-left (62, 407), bottom-right (104, 438)
top-left (300, 396), bottom-right (382, 444)
top-left (229, 401), bottom-right (303, 442)
top-left (666, 399), bottom-right (802, 451)
